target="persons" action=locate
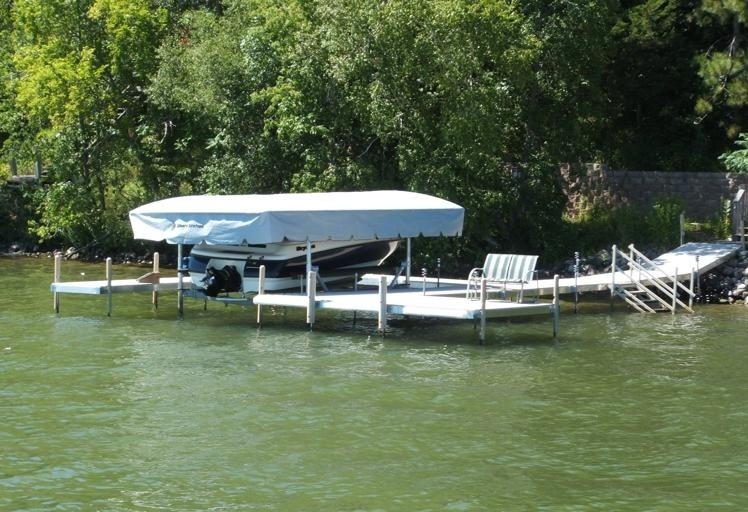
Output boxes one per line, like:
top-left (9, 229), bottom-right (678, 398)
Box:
top-left (197, 263), bottom-right (242, 297)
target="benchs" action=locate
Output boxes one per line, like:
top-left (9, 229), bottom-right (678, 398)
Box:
top-left (465, 253), bottom-right (539, 302)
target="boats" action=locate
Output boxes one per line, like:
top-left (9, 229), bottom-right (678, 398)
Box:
top-left (189, 237), bottom-right (402, 295)
